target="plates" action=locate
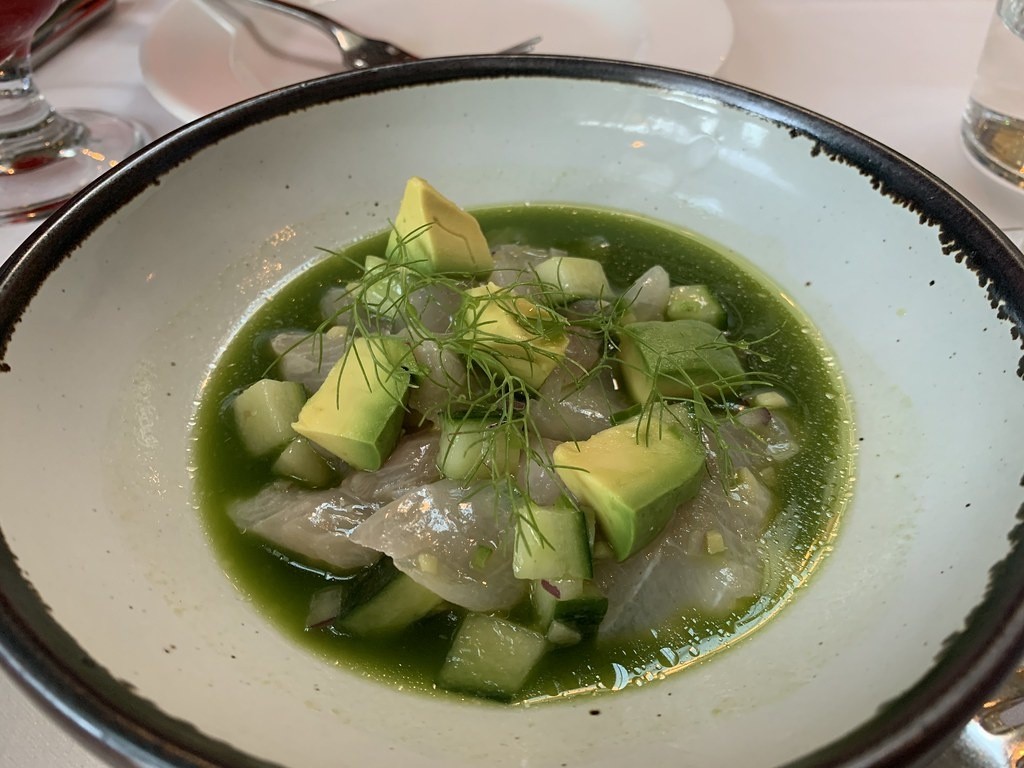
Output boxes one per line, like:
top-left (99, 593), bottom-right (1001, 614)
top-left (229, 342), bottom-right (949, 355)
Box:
top-left (137, 0), bottom-right (737, 124)
top-left (0, 52), bottom-right (1024, 768)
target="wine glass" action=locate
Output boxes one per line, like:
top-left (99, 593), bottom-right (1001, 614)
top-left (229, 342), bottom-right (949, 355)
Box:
top-left (0, 0), bottom-right (154, 228)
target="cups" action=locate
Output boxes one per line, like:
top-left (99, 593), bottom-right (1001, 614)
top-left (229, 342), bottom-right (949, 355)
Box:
top-left (957, 0), bottom-right (1024, 196)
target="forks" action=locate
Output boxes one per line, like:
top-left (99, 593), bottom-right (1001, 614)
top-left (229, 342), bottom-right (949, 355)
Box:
top-left (243, 0), bottom-right (542, 71)
